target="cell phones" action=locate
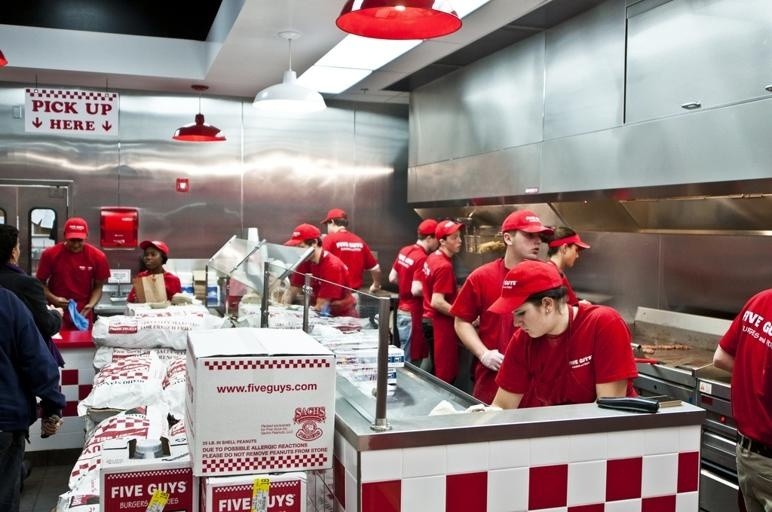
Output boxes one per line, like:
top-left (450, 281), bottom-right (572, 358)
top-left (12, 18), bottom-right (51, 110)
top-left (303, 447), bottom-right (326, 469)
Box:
top-left (596, 397), bottom-right (659, 412)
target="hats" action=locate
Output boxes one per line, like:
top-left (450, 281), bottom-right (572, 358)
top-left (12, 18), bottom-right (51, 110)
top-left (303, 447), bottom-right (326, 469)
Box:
top-left (502, 209), bottom-right (555, 236)
top-left (320, 208), bottom-right (348, 225)
top-left (139, 239), bottom-right (169, 257)
top-left (435, 220), bottom-right (465, 241)
top-left (284, 223), bottom-right (321, 246)
top-left (486, 258), bottom-right (565, 316)
top-left (548, 232), bottom-right (591, 252)
top-left (64, 216), bottom-right (89, 241)
top-left (417, 218), bottom-right (438, 235)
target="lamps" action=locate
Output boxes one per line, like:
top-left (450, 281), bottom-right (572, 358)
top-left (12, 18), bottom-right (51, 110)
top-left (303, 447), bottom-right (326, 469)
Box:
top-left (252, 30), bottom-right (327, 118)
top-left (173, 85), bottom-right (226, 143)
top-left (336, 0), bottom-right (463, 39)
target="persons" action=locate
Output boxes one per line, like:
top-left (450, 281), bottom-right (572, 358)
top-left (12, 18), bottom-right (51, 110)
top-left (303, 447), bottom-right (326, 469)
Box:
top-left (281, 223), bottom-right (357, 317)
top-left (0, 288), bottom-right (66, 512)
top-left (320, 210), bottom-right (385, 318)
top-left (35, 216), bottom-right (112, 331)
top-left (126, 240), bottom-right (181, 302)
top-left (712, 287), bottom-right (772, 511)
top-left (412, 220), bottom-right (466, 385)
top-left (388, 218), bottom-right (442, 367)
top-left (451, 210), bottom-right (591, 404)
top-left (488, 260), bottom-right (639, 409)
top-left (0, 223), bottom-right (64, 480)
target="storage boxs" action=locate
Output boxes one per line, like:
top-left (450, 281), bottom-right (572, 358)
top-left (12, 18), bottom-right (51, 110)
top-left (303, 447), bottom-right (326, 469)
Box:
top-left (200, 472), bottom-right (308, 512)
top-left (99, 439), bottom-right (200, 512)
top-left (185, 328), bottom-right (335, 476)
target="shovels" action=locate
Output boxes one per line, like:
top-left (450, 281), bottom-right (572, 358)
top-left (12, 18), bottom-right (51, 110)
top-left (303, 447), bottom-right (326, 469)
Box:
top-left (634, 354), bottom-right (693, 368)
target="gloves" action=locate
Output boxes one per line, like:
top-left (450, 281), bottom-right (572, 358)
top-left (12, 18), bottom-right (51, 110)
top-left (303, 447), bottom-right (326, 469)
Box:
top-left (481, 348), bottom-right (505, 372)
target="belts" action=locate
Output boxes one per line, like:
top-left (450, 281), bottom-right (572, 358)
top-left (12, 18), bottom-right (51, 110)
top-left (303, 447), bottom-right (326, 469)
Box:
top-left (736, 431), bottom-right (772, 459)
